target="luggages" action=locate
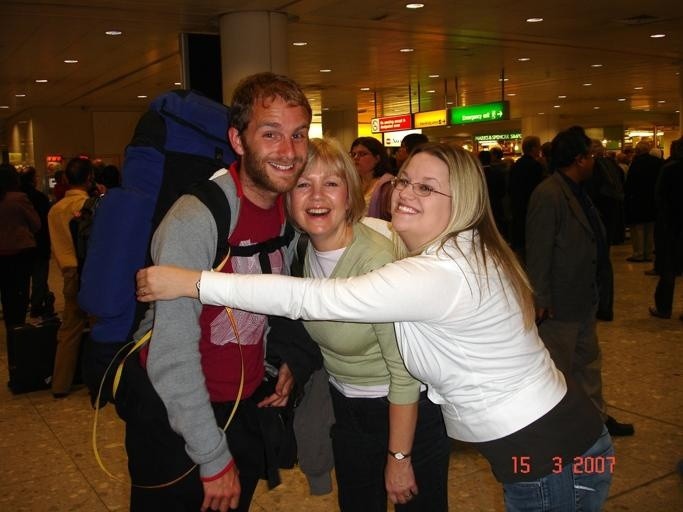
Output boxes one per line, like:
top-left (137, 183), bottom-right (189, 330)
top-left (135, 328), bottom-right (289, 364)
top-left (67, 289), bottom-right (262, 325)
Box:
top-left (8, 309), bottom-right (62, 395)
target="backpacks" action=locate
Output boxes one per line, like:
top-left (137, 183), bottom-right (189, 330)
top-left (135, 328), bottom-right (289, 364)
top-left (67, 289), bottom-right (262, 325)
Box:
top-left (77, 87), bottom-right (297, 411)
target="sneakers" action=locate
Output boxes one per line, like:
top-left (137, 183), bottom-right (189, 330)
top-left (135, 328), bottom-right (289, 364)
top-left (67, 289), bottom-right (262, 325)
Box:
top-left (596, 312), bottom-right (613, 321)
top-left (648, 306), bottom-right (672, 319)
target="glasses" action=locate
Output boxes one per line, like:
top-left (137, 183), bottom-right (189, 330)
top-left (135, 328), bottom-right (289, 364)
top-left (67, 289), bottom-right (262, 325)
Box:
top-left (390, 177), bottom-right (451, 201)
top-left (347, 151), bottom-right (370, 158)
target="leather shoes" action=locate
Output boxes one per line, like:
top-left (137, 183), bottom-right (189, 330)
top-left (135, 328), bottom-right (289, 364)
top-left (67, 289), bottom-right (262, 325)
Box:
top-left (607, 415), bottom-right (635, 437)
